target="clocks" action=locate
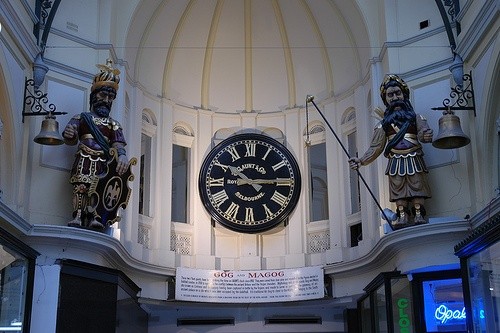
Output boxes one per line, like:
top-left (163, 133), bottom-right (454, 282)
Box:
top-left (199, 133), bottom-right (302, 233)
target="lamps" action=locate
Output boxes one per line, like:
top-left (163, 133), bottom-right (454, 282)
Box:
top-left (22, 76), bottom-right (69, 145)
top-left (381, 208), bottom-right (397, 234)
top-left (431, 72), bottom-right (479, 150)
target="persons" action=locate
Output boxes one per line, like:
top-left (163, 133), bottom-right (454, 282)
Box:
top-left (346, 74), bottom-right (433, 229)
top-left (62, 63), bottom-right (130, 231)
